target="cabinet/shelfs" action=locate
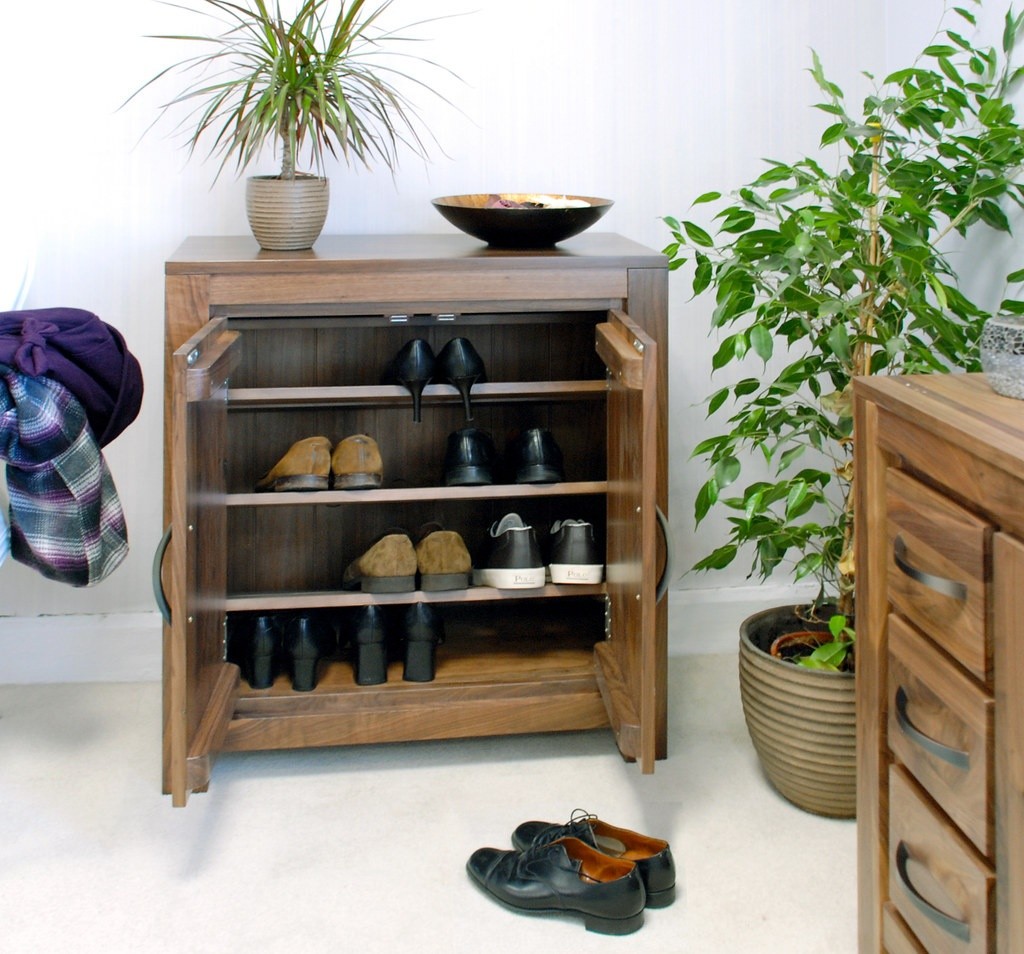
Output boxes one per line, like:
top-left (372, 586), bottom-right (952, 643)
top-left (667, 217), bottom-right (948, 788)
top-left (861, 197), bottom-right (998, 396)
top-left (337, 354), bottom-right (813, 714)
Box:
top-left (847, 367), bottom-right (1024, 954)
top-left (150, 229), bottom-right (673, 807)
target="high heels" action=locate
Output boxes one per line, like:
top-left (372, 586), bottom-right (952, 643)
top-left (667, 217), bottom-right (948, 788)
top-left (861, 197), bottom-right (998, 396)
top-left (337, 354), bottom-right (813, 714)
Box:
top-left (285, 615), bottom-right (337, 692)
top-left (246, 612), bottom-right (285, 689)
top-left (435, 336), bottom-right (487, 423)
top-left (381, 337), bottom-right (435, 425)
top-left (344, 605), bottom-right (392, 685)
top-left (404, 600), bottom-right (443, 682)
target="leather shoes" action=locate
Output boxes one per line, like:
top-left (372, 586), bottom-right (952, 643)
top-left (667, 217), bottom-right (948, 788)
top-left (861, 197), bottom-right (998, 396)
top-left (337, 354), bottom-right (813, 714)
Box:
top-left (467, 836), bottom-right (646, 934)
top-left (513, 823), bottom-right (676, 909)
top-left (505, 428), bottom-right (565, 484)
top-left (442, 428), bottom-right (493, 485)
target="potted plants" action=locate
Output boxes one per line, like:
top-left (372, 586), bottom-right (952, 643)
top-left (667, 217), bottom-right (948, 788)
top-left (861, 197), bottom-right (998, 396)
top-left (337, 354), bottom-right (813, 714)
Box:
top-left (657, 0), bottom-right (1024, 818)
top-left (111, 0), bottom-right (475, 250)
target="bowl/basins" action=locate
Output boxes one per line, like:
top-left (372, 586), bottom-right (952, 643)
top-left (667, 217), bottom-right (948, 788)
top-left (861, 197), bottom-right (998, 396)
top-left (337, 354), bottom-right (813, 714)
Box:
top-left (431, 194), bottom-right (617, 252)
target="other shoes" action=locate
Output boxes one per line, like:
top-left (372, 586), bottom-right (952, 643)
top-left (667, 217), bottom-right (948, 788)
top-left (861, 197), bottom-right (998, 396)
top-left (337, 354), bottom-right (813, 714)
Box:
top-left (332, 433), bottom-right (384, 490)
top-left (342, 528), bottom-right (418, 593)
top-left (257, 436), bottom-right (333, 490)
top-left (549, 520), bottom-right (604, 585)
top-left (472, 512), bottom-right (545, 588)
top-left (414, 521), bottom-right (471, 590)
